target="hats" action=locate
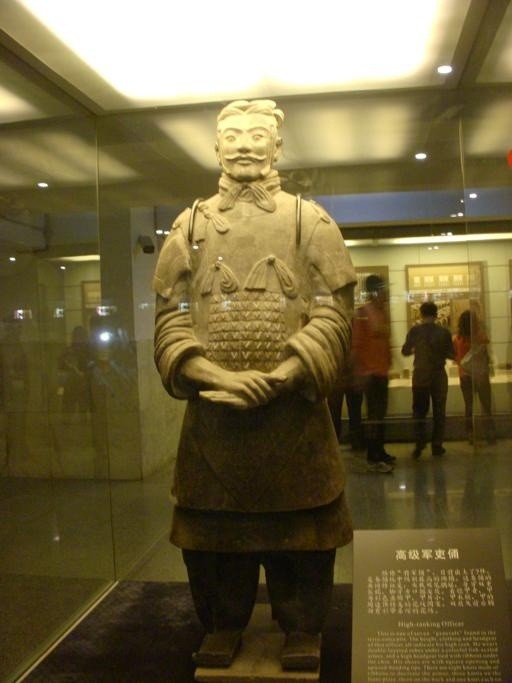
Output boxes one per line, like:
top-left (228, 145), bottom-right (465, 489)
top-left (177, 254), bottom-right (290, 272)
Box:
top-left (366, 275), bottom-right (389, 292)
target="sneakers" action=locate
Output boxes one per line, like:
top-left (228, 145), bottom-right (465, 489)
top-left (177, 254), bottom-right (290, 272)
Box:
top-left (367, 450), bottom-right (396, 473)
top-left (412, 441), bottom-right (427, 459)
top-left (431, 445), bottom-right (446, 457)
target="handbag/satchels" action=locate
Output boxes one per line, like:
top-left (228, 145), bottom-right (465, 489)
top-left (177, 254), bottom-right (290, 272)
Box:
top-left (461, 344), bottom-right (490, 381)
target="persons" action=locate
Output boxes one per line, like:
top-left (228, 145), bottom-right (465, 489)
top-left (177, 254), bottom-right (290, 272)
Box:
top-left (328, 341), bottom-right (367, 453)
top-left (450, 310), bottom-right (498, 446)
top-left (2, 260), bottom-right (106, 485)
top-left (348, 272), bottom-right (396, 475)
top-left (148, 98), bottom-right (361, 671)
top-left (400, 301), bottom-right (456, 457)
